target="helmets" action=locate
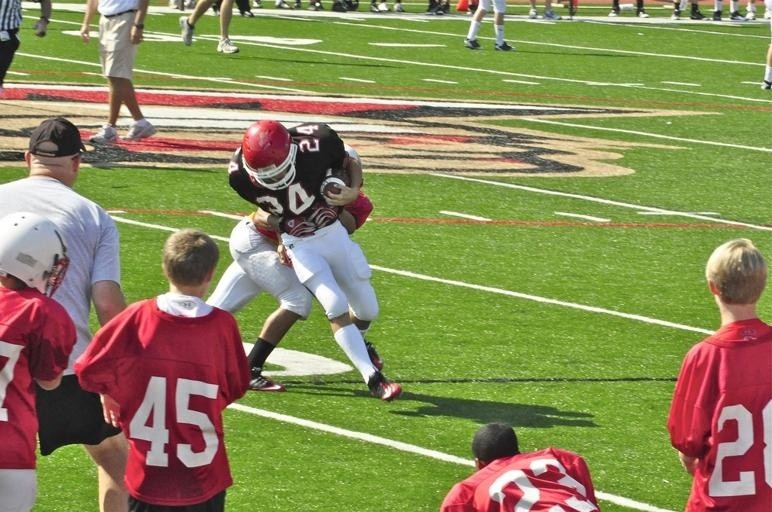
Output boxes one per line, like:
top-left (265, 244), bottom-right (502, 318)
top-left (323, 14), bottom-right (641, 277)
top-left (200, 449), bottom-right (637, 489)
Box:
top-left (0, 213), bottom-right (69, 297)
top-left (242, 120), bottom-right (298, 177)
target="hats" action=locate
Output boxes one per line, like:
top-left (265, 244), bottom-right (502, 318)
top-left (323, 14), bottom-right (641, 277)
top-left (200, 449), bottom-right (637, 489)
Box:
top-left (29, 119), bottom-right (85, 158)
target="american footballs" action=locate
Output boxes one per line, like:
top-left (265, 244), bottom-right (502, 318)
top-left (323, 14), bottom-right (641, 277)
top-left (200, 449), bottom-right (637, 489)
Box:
top-left (322, 176), bottom-right (351, 198)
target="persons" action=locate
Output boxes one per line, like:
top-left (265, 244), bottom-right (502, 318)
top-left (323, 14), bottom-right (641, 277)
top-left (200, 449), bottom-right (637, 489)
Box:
top-left (439, 420), bottom-right (604, 512)
top-left (170, 1), bottom-right (772, 54)
top-left (204, 141), bottom-right (376, 393)
top-left (0, 1), bottom-right (53, 94)
top-left (664, 236), bottom-right (772, 512)
top-left (0, 113), bottom-right (130, 511)
top-left (80, 0), bottom-right (157, 144)
top-left (70, 228), bottom-right (252, 510)
top-left (226, 118), bottom-right (404, 402)
top-left (1, 211), bottom-right (78, 512)
top-left (759, 41), bottom-right (772, 90)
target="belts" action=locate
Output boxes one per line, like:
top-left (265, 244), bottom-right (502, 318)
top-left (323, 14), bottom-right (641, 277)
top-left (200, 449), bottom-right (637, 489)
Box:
top-left (104, 9), bottom-right (136, 18)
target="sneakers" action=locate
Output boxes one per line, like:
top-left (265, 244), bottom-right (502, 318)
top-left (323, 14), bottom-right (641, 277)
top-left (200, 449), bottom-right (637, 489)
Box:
top-left (247, 341), bottom-right (401, 400)
top-left (88, 124), bottom-right (117, 144)
top-left (761, 80), bottom-right (772, 90)
top-left (119, 121), bottom-right (156, 141)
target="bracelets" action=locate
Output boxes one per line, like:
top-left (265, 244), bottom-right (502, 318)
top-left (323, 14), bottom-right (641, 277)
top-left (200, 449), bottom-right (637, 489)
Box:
top-left (41, 15), bottom-right (49, 23)
top-left (132, 22), bottom-right (145, 30)
